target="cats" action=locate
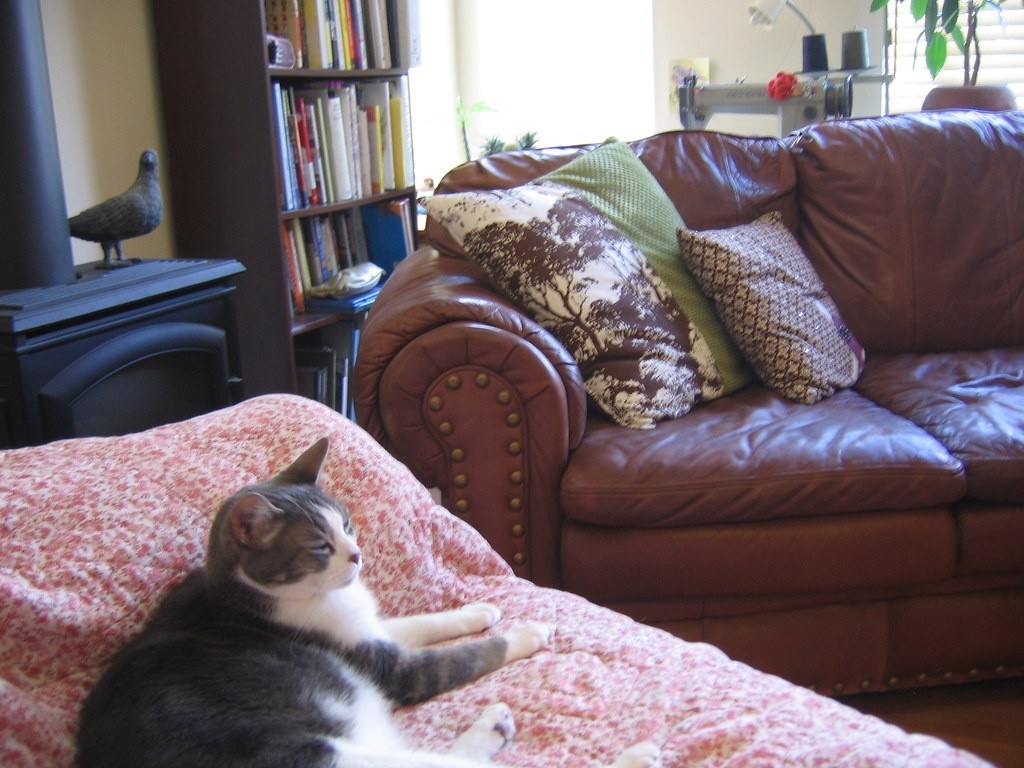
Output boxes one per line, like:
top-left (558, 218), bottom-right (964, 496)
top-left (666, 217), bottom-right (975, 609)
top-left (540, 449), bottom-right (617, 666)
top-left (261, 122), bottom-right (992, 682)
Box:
top-left (75, 439), bottom-right (547, 768)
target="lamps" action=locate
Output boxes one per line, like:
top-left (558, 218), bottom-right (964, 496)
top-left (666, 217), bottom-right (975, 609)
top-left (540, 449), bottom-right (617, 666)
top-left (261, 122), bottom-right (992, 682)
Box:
top-left (748, 0), bottom-right (816, 34)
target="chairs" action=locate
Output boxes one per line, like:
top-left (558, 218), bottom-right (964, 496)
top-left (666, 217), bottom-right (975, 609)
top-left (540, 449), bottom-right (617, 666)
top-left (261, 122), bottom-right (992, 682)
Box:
top-left (0, 391), bottom-right (1000, 768)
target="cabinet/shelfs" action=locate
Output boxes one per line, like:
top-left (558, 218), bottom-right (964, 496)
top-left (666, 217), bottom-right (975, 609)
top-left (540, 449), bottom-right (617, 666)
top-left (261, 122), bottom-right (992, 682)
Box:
top-left (148, 0), bottom-right (421, 405)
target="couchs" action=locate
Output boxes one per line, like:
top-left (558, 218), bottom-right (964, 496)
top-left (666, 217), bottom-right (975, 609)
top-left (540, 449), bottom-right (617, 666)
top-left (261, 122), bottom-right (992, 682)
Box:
top-left (351, 106), bottom-right (1024, 696)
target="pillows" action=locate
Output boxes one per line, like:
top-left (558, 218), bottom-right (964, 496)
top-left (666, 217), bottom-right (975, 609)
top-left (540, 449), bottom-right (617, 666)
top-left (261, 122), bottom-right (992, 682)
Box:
top-left (675, 212), bottom-right (865, 405)
top-left (416, 136), bottom-right (751, 430)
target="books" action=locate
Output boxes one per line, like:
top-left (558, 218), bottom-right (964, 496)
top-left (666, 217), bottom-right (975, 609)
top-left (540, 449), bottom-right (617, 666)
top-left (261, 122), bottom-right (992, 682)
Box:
top-left (297, 311), bottom-right (373, 423)
top-left (265, 0), bottom-right (416, 316)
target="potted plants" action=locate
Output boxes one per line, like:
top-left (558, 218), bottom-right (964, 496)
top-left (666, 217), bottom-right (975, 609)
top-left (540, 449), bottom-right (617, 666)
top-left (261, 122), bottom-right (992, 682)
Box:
top-left (870, 1), bottom-right (1017, 112)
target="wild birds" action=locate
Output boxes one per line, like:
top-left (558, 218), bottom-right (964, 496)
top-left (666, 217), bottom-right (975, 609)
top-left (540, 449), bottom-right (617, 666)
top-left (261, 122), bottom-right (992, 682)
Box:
top-left (67, 149), bottom-right (164, 269)
